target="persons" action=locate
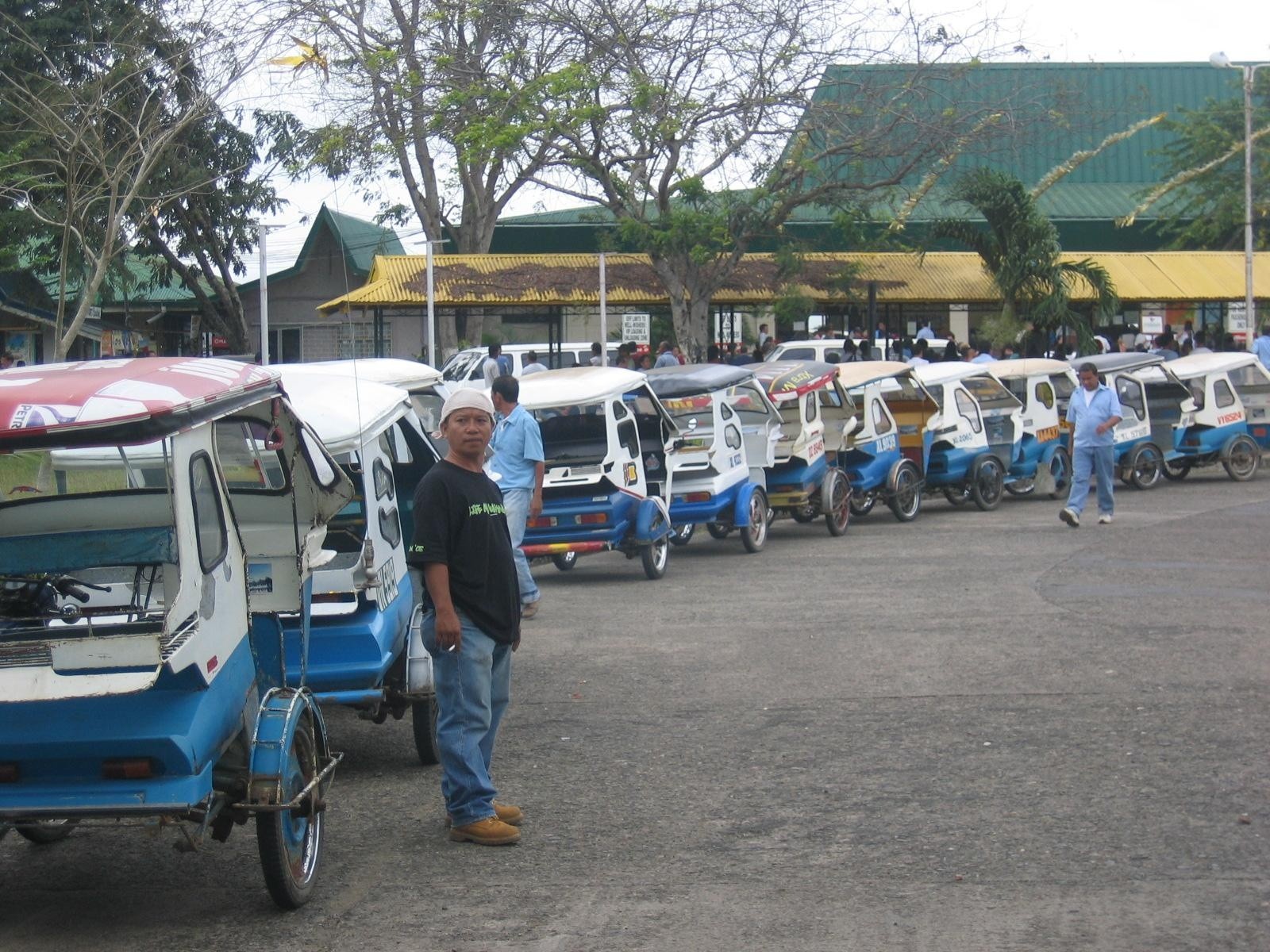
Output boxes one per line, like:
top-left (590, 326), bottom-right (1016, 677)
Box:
top-left (653, 341), bottom-right (685, 370)
top-left (888, 333), bottom-right (939, 365)
top-left (758, 322), bottom-right (769, 349)
top-left (943, 319), bottom-right (1068, 362)
top-left (520, 351), bottom-right (548, 375)
top-left (482, 344), bottom-right (500, 388)
top-left (916, 319), bottom-right (935, 339)
top-left (614, 340), bottom-right (652, 372)
top-left (707, 342), bottom-right (763, 367)
top-left (1108, 319), bottom-right (1270, 372)
top-left (589, 341), bottom-right (611, 366)
top-left (1058, 362), bottom-right (1123, 526)
top-left (480, 375), bottom-right (545, 619)
top-left (839, 338), bottom-right (881, 361)
top-left (405, 388), bottom-right (525, 845)
top-left (813, 321), bottom-right (901, 339)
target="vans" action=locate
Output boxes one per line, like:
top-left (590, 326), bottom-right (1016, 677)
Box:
top-left (441, 342), bottom-right (650, 401)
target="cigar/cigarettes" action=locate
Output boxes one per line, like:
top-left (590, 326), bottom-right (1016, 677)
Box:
top-left (449, 645), bottom-right (455, 652)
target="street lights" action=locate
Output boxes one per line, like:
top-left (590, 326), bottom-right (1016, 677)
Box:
top-left (1209, 51), bottom-right (1269, 385)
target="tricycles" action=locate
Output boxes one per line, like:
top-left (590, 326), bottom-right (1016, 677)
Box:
top-left (479, 367), bottom-right (696, 579)
top-left (1, 358), bottom-right (355, 912)
top-left (250, 358), bottom-right (495, 765)
top-left (644, 363), bottom-right (785, 553)
top-left (740, 339), bottom-right (1270, 536)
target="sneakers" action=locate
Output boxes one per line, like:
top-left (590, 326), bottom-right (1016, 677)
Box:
top-left (1060, 508), bottom-right (1079, 526)
top-left (1099, 513), bottom-right (1112, 525)
top-left (522, 593), bottom-right (540, 618)
top-left (445, 796), bottom-right (522, 825)
top-left (450, 816), bottom-right (520, 844)
